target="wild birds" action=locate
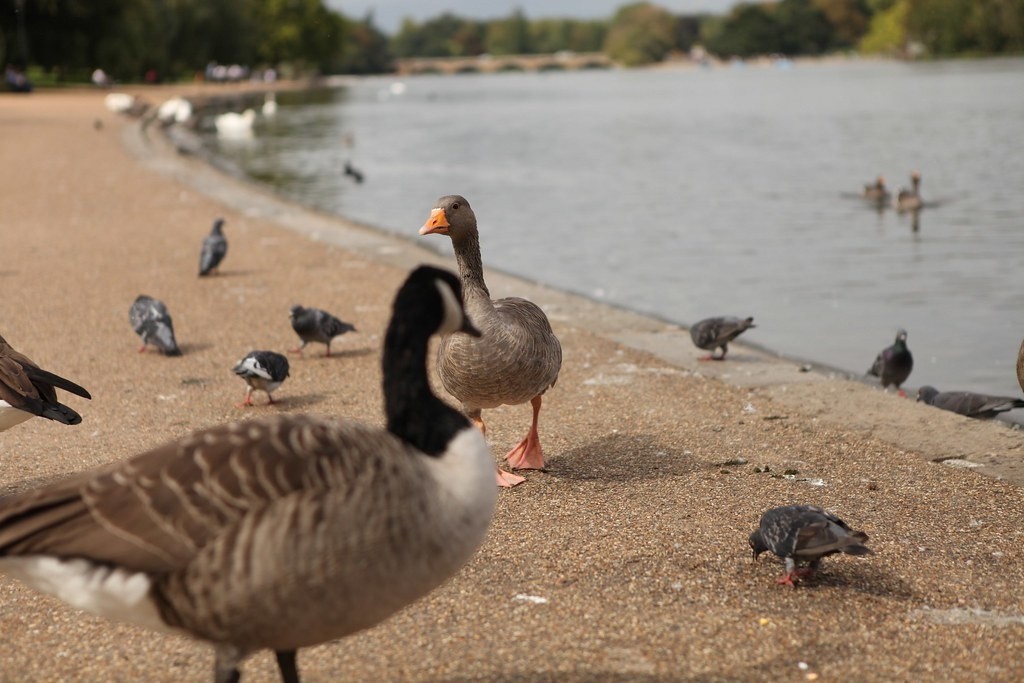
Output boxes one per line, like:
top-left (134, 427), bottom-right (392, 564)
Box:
top-left (104, 92), bottom-right (139, 112)
top-left (286, 306), bottom-right (355, 356)
top-left (867, 329), bottom-right (913, 396)
top-left (230, 350), bottom-right (290, 406)
top-left (418, 196), bottom-right (563, 473)
top-left (687, 315), bottom-right (755, 360)
top-left (213, 109), bottom-right (257, 131)
top-left (197, 218), bottom-right (228, 276)
top-left (0, 265), bottom-right (499, 683)
top-left (0, 337), bottom-right (91, 432)
top-left (865, 178), bottom-right (887, 198)
top-left (748, 506), bottom-right (875, 587)
top-left (128, 294), bottom-right (182, 356)
top-left (157, 97), bottom-right (192, 124)
top-left (897, 171), bottom-right (924, 206)
top-left (916, 385), bottom-right (1024, 420)
